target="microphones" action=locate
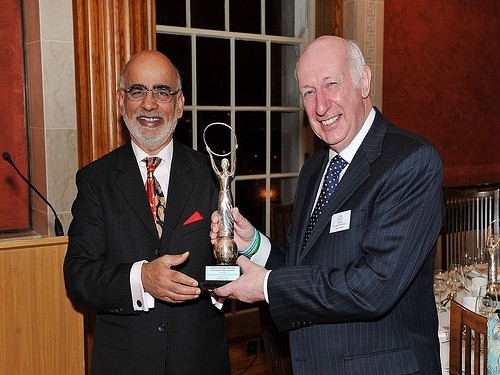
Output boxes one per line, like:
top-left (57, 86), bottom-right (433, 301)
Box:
top-left (2, 152), bottom-right (64, 236)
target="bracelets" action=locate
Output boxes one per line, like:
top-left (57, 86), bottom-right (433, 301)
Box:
top-left (237, 228), bottom-right (260, 259)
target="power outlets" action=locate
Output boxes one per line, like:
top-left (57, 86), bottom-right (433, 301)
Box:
top-left (246, 343), bottom-right (258, 358)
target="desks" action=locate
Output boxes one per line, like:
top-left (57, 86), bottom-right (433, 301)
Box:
top-left (434, 263), bottom-right (500, 375)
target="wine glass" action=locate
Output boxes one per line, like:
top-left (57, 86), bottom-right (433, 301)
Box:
top-left (475, 296), bottom-right (493, 350)
top-left (435, 270), bottom-right (447, 312)
top-left (447, 264), bottom-right (464, 298)
top-left (474, 248), bottom-right (488, 278)
top-left (459, 252), bottom-right (474, 280)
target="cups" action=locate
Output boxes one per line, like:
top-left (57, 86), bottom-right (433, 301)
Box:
top-left (479, 286), bottom-right (497, 310)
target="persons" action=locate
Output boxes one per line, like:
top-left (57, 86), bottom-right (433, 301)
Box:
top-left (63, 50), bottom-right (230, 375)
top-left (211, 36), bottom-right (443, 375)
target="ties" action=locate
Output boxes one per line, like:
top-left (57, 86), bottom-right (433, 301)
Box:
top-left (301, 155), bottom-right (350, 254)
top-left (141, 156), bottom-right (167, 240)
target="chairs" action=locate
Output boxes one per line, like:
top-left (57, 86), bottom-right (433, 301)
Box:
top-left (450, 298), bottom-right (489, 375)
top-left (259, 203), bottom-right (294, 375)
top-left (441, 186), bottom-right (500, 270)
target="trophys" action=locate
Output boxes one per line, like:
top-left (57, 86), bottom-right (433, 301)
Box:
top-left (484, 219), bottom-right (500, 307)
top-left (203, 122), bottom-right (241, 288)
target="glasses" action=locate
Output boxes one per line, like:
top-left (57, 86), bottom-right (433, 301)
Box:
top-left (121, 86), bottom-right (179, 103)
